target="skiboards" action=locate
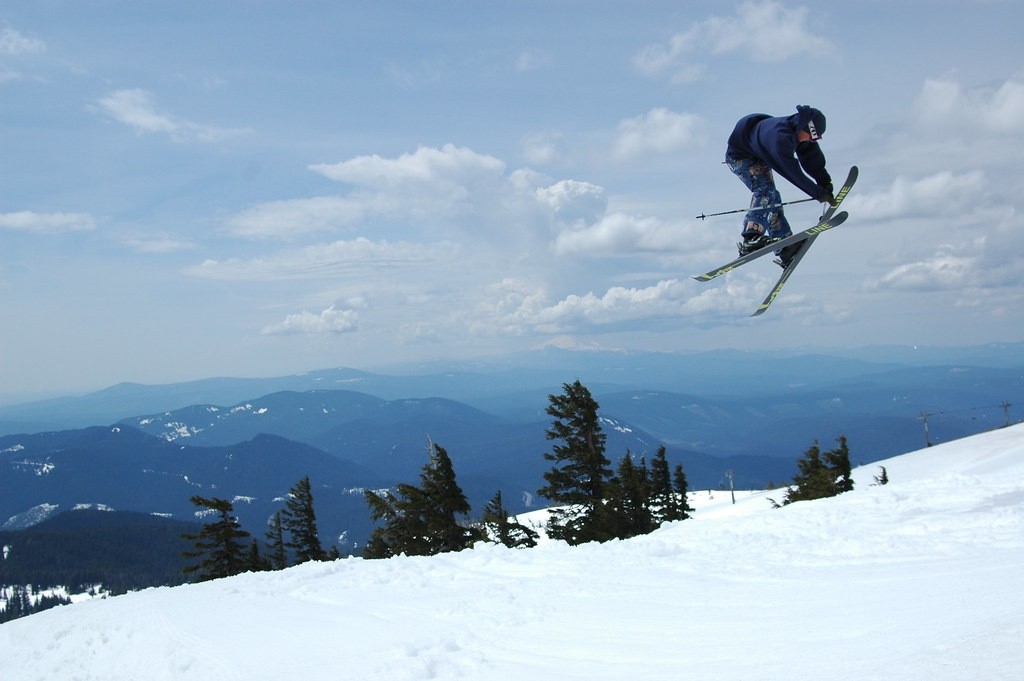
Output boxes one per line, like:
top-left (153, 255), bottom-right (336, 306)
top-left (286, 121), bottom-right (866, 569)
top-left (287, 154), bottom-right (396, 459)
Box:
top-left (689, 165), bottom-right (859, 318)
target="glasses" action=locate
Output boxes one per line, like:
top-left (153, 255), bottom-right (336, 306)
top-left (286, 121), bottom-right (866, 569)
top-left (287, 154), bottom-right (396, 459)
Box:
top-left (812, 137), bottom-right (822, 141)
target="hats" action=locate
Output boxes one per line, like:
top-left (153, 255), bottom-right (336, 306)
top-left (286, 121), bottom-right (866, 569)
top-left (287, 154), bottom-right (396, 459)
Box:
top-left (802, 108), bottom-right (826, 136)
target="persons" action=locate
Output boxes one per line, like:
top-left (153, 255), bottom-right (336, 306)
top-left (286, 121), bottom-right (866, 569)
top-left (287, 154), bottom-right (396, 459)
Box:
top-left (723, 103), bottom-right (840, 271)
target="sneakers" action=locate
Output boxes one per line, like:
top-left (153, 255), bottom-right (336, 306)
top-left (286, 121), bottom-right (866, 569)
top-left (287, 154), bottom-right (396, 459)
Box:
top-left (778, 235), bottom-right (808, 265)
top-left (739, 232), bottom-right (783, 257)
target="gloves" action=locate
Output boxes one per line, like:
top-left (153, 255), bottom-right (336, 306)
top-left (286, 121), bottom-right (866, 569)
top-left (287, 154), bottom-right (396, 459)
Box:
top-left (821, 180), bottom-right (833, 193)
top-left (817, 186), bottom-right (834, 203)
